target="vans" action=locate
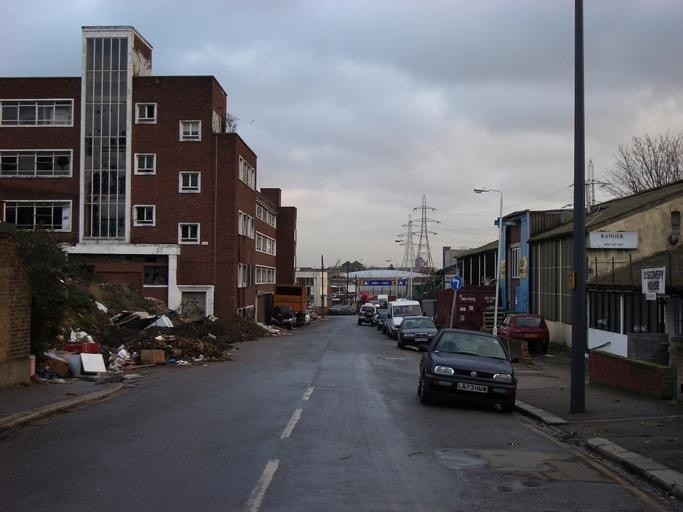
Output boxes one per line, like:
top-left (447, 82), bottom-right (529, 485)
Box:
top-left (269, 305), bottom-right (297, 331)
top-left (387, 298), bottom-right (422, 340)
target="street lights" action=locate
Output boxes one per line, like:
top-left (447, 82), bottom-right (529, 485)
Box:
top-left (472, 185), bottom-right (503, 337)
top-left (395, 239), bottom-right (412, 312)
top-left (385, 259), bottom-right (397, 299)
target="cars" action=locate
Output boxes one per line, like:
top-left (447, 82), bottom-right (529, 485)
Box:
top-left (395, 316), bottom-right (440, 350)
top-left (498, 313), bottom-right (550, 355)
top-left (414, 326), bottom-right (516, 412)
top-left (372, 266), bottom-right (417, 328)
top-left (328, 285), bottom-right (356, 316)
top-left (303, 310), bottom-right (310, 326)
top-left (357, 300), bottom-right (386, 331)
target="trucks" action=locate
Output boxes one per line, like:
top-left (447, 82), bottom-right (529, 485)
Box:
top-left (432, 286), bottom-right (501, 332)
top-left (273, 284), bottom-right (307, 326)
